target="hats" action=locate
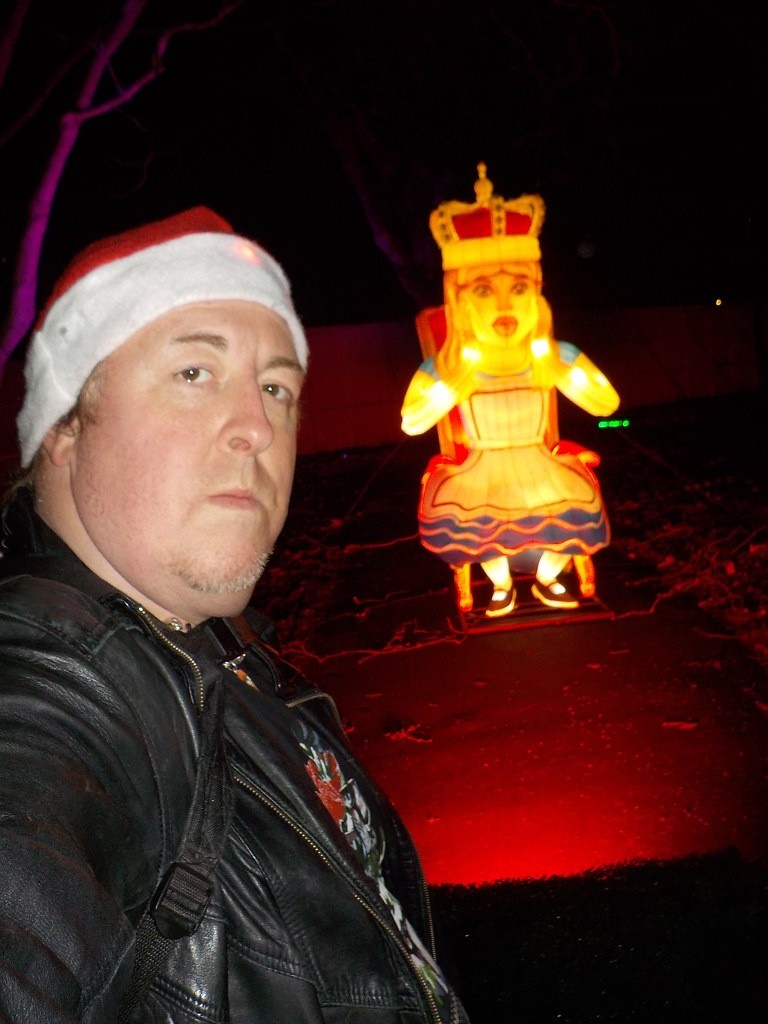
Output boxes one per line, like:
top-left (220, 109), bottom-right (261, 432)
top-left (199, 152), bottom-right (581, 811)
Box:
top-left (16, 206), bottom-right (310, 470)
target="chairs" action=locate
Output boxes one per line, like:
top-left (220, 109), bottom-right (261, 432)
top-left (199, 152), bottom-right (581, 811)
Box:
top-left (416, 302), bottom-right (601, 608)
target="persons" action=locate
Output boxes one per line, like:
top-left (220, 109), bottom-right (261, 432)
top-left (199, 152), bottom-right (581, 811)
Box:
top-left (0, 208), bottom-right (467, 1024)
top-left (401, 163), bottom-right (620, 616)
